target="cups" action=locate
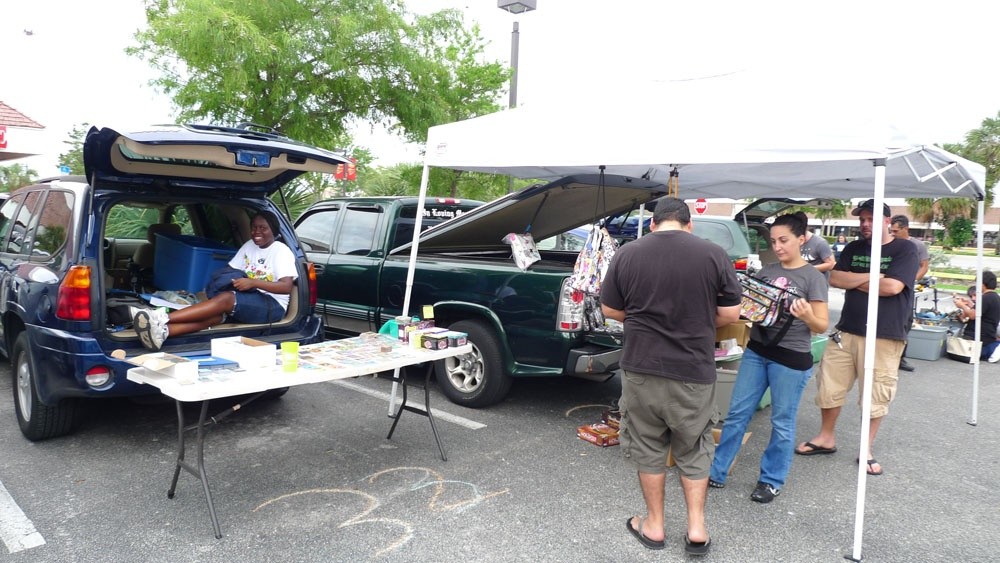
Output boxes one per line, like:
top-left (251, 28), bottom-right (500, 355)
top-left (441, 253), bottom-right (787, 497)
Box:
top-left (281, 341), bottom-right (299, 371)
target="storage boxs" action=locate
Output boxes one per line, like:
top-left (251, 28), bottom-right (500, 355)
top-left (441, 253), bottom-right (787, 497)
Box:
top-left (666, 320), bottom-right (829, 477)
top-left (576, 407), bottom-right (621, 448)
top-left (905, 318), bottom-right (965, 361)
top-left (152, 231), bottom-right (239, 294)
top-left (211, 336), bottom-right (276, 372)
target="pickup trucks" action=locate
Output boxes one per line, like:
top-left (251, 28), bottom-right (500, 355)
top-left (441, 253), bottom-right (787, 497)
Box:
top-left (277, 169), bottom-right (675, 412)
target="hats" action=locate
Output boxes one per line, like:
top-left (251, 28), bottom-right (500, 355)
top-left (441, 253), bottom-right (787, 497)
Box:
top-left (852, 199), bottom-right (890, 220)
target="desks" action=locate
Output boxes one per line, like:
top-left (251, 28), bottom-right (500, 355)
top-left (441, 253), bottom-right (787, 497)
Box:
top-left (127, 332), bottom-right (473, 539)
top-left (913, 287), bottom-right (939, 318)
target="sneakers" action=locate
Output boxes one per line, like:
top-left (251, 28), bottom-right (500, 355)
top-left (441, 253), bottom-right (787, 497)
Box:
top-left (128, 305), bottom-right (170, 327)
top-left (134, 311), bottom-right (167, 352)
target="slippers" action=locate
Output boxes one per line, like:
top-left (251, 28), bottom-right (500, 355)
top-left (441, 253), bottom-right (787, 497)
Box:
top-left (626, 516), bottom-right (665, 550)
top-left (857, 457), bottom-right (883, 475)
top-left (684, 532), bottom-right (712, 554)
top-left (794, 441), bottom-right (836, 455)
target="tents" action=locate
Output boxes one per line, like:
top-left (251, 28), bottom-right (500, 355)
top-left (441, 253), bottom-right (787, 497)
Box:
top-left (387, 70), bottom-right (987, 563)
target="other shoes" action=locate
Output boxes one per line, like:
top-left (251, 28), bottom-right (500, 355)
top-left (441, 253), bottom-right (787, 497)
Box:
top-left (899, 356), bottom-right (914, 371)
top-left (707, 478), bottom-right (724, 488)
top-left (988, 343), bottom-right (1000, 363)
top-left (751, 480), bottom-right (780, 502)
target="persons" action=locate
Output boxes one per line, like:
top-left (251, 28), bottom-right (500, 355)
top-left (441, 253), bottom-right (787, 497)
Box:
top-left (954, 270), bottom-right (1000, 364)
top-left (832, 233), bottom-right (848, 263)
top-left (795, 211), bottom-right (836, 287)
top-left (599, 197), bottom-right (741, 556)
top-left (888, 214), bottom-right (928, 373)
top-left (795, 199), bottom-right (918, 475)
top-left (128, 211), bottom-right (298, 352)
top-left (706, 212), bottom-right (829, 504)
top-left (967, 285), bottom-right (976, 304)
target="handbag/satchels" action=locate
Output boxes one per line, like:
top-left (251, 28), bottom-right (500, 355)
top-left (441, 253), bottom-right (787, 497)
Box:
top-left (734, 272), bottom-right (803, 355)
top-left (568, 223), bottom-right (624, 333)
top-left (501, 232), bottom-right (542, 271)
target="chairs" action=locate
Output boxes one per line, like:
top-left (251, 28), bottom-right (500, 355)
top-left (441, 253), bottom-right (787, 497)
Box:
top-left (125, 224), bottom-right (181, 292)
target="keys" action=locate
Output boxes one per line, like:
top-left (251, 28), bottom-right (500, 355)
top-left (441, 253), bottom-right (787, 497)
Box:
top-left (833, 335), bottom-right (844, 350)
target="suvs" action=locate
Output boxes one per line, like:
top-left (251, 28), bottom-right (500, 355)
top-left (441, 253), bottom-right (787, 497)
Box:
top-left (659, 193), bottom-right (823, 279)
top-left (552, 208), bottom-right (653, 253)
top-left (0, 120), bottom-right (354, 442)
